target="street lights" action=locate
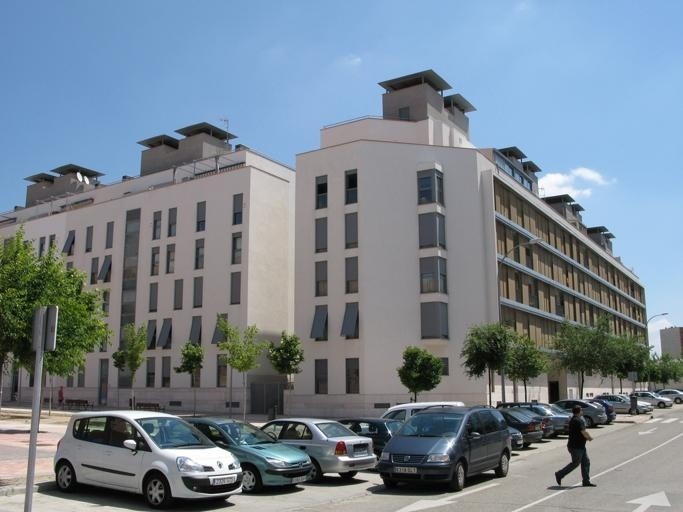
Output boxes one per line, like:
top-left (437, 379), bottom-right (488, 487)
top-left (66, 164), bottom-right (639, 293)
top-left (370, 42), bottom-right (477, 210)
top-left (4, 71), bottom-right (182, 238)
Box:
top-left (644, 312), bottom-right (668, 391)
top-left (498, 235), bottom-right (543, 408)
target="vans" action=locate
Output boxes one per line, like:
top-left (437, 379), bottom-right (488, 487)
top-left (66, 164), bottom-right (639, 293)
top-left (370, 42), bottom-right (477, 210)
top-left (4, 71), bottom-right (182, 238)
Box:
top-left (375, 404), bottom-right (511, 491)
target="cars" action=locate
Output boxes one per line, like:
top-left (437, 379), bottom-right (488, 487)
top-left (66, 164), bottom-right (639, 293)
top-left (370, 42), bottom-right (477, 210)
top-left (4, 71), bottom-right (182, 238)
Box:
top-left (494, 388), bottom-right (682, 452)
top-left (155, 416), bottom-right (313, 492)
top-left (53, 409), bottom-right (243, 509)
top-left (243, 417), bottom-right (377, 484)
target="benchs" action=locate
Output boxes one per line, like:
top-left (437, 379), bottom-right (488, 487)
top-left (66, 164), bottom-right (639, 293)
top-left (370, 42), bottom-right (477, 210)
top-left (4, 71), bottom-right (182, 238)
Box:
top-left (62, 399), bottom-right (95, 410)
top-left (135, 402), bottom-right (165, 412)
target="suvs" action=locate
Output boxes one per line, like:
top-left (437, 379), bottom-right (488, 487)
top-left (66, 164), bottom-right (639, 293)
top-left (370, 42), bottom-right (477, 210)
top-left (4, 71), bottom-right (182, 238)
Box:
top-left (319, 417), bottom-right (416, 474)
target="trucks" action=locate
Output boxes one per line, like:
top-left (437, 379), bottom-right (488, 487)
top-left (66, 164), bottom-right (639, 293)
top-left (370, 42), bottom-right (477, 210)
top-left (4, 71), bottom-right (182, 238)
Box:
top-left (357, 401), bottom-right (465, 435)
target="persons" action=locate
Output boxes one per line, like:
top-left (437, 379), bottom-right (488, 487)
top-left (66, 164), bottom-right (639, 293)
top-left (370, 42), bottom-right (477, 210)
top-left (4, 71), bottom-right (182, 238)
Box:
top-left (58, 387), bottom-right (64, 406)
top-left (555, 405), bottom-right (596, 487)
